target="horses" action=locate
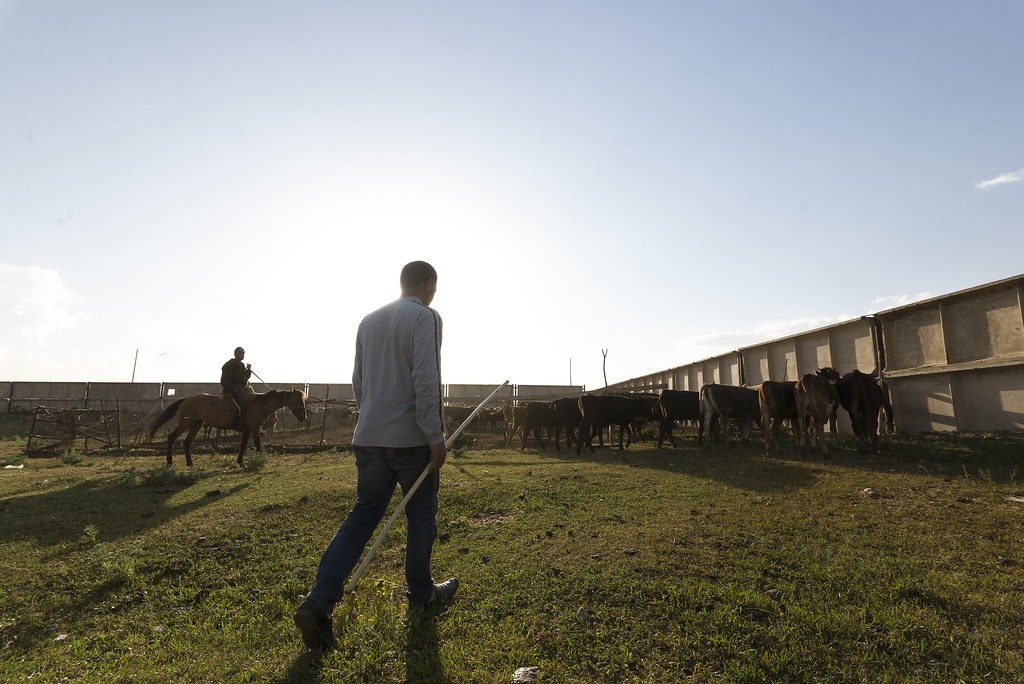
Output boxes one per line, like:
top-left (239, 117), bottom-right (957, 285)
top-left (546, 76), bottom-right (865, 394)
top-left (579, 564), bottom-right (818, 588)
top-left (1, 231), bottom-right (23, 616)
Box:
top-left (445, 367), bottom-right (897, 454)
top-left (146, 385), bottom-right (307, 469)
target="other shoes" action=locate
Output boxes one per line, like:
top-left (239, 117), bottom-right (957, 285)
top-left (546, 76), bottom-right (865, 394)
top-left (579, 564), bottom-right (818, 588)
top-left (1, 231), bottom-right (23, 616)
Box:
top-left (408, 576), bottom-right (459, 611)
top-left (293, 596), bottom-right (333, 649)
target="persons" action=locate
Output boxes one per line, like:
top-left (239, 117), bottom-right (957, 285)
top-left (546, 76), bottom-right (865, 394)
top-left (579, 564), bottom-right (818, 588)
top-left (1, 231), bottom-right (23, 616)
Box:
top-left (292, 259), bottom-right (460, 650)
top-left (221, 347), bottom-right (252, 432)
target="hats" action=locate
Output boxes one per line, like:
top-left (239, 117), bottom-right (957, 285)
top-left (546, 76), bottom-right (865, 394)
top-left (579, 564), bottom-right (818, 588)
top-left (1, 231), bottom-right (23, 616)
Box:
top-left (234, 347), bottom-right (245, 353)
